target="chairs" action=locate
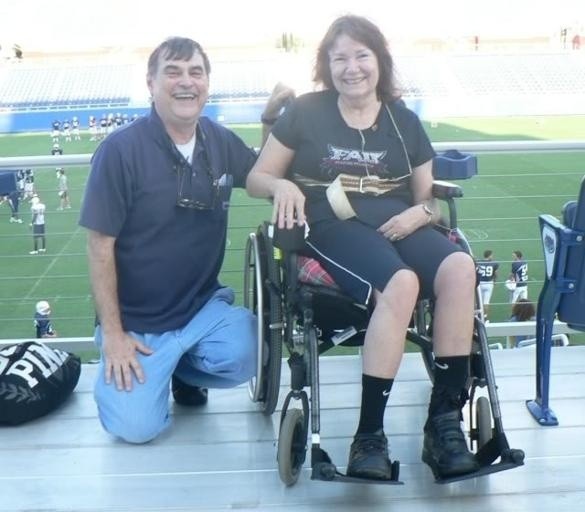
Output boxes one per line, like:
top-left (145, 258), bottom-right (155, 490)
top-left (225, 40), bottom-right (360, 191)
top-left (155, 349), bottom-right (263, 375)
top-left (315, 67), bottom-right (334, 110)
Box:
top-left (524, 173), bottom-right (585, 424)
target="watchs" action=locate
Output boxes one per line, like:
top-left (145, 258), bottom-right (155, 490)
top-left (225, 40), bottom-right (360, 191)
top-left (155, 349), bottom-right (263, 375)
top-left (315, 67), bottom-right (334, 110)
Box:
top-left (419, 199), bottom-right (435, 226)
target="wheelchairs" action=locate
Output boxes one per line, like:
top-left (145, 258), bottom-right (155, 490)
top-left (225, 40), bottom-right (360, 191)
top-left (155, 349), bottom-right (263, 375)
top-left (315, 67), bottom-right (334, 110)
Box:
top-left (243, 82), bottom-right (527, 488)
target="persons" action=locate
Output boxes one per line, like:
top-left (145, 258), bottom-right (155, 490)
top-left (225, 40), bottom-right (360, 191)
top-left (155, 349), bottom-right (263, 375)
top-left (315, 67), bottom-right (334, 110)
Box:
top-left (475, 250), bottom-right (499, 322)
top-left (51, 114), bottom-right (141, 176)
top-left (34, 300), bottom-right (54, 338)
top-left (505, 299), bottom-right (535, 348)
top-left (79, 37), bottom-right (299, 444)
top-left (246, 15), bottom-right (480, 480)
top-left (29, 197), bottom-right (46, 254)
top-left (55, 168), bottom-right (71, 211)
top-left (509, 250), bottom-right (528, 304)
top-left (0, 165), bottom-right (34, 222)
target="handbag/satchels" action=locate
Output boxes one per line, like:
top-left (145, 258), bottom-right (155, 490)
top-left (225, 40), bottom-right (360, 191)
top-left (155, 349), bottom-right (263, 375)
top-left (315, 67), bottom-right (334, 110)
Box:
top-left (0, 341), bottom-right (80, 426)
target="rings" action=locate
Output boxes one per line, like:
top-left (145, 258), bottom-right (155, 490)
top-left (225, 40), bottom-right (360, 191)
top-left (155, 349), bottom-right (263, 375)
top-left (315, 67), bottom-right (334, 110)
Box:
top-left (393, 234), bottom-right (399, 239)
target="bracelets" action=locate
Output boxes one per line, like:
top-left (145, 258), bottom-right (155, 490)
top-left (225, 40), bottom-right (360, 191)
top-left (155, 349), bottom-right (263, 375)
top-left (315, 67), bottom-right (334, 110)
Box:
top-left (259, 115), bottom-right (279, 126)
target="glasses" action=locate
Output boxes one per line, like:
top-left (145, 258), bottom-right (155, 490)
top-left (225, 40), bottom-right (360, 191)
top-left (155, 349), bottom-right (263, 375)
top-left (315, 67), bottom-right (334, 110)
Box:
top-left (177, 156), bottom-right (220, 210)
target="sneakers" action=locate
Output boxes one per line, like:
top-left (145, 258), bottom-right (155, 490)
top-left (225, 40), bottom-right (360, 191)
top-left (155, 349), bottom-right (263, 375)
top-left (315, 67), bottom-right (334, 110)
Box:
top-left (421, 413), bottom-right (480, 478)
top-left (172, 377), bottom-right (207, 405)
top-left (348, 433), bottom-right (392, 480)
